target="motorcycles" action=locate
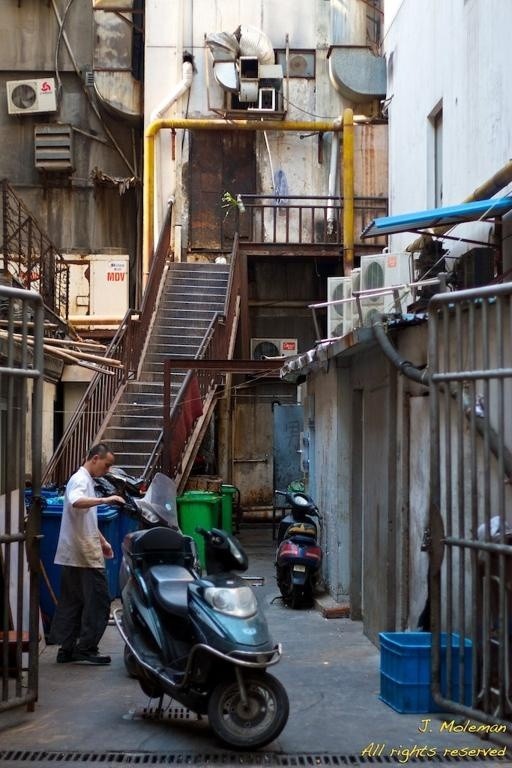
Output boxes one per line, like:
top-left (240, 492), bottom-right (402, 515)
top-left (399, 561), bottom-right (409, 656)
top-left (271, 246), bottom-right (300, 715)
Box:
top-left (272, 477), bottom-right (325, 610)
top-left (112, 523), bottom-right (292, 752)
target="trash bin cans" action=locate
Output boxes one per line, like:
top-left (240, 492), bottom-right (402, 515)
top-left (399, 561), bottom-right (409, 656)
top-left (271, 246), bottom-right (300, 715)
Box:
top-left (176, 484), bottom-right (236, 569)
top-left (25, 488), bottom-right (139, 618)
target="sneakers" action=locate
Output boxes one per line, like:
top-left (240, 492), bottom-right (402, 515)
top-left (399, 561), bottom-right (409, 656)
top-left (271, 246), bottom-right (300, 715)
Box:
top-left (55, 644), bottom-right (112, 664)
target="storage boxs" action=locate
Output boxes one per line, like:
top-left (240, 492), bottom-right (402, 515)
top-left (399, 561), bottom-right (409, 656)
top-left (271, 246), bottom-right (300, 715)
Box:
top-left (377, 632), bottom-right (472, 715)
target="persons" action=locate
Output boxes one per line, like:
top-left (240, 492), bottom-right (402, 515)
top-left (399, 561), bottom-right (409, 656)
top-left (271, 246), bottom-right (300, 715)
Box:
top-left (54, 443), bottom-right (127, 668)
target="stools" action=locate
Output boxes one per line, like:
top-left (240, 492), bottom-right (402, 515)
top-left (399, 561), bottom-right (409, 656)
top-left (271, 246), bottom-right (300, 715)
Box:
top-left (0, 630), bottom-right (41, 687)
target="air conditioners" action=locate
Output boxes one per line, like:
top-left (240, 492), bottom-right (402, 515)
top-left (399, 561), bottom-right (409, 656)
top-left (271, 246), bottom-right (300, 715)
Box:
top-left (6, 77), bottom-right (57, 115)
top-left (327, 252), bottom-right (415, 339)
top-left (0, 254), bottom-right (129, 331)
top-left (250, 338), bottom-right (298, 361)
